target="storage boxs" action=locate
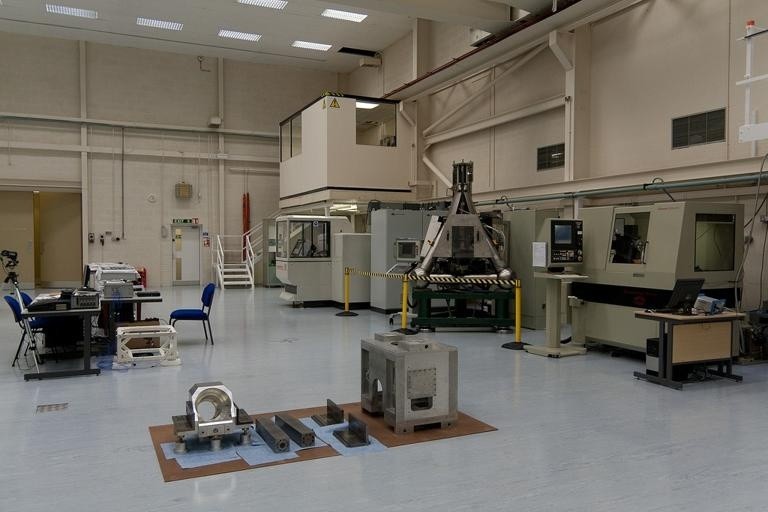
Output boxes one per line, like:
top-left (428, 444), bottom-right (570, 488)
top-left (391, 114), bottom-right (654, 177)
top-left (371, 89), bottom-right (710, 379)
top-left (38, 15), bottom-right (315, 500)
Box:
top-left (113, 318), bottom-right (160, 348)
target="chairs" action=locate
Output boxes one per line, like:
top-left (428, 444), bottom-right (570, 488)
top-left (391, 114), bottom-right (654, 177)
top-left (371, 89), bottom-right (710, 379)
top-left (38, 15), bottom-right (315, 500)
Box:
top-left (169, 283), bottom-right (215, 345)
top-left (3, 296), bottom-right (58, 367)
top-left (16, 288), bottom-right (57, 356)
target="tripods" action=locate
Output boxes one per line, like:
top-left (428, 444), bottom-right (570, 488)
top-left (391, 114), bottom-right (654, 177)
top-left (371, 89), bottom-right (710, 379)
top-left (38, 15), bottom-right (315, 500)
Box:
top-left (2, 282), bottom-right (42, 374)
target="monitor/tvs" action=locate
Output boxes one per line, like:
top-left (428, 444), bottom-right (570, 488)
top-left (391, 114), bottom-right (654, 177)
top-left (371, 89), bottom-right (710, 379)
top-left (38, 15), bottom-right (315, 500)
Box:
top-left (667, 278), bottom-right (705, 315)
top-left (83, 265), bottom-right (91, 291)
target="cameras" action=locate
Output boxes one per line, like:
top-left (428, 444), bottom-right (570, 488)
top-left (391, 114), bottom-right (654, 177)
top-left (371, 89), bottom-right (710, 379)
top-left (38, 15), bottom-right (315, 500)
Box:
top-left (0, 250), bottom-right (17, 261)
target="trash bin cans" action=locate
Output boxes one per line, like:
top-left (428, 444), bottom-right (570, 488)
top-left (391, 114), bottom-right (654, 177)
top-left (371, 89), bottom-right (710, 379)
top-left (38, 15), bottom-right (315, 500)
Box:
top-left (646, 336), bottom-right (690, 383)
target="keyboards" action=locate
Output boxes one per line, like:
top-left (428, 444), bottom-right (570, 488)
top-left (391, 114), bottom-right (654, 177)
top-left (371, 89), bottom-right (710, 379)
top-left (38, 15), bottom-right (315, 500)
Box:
top-left (651, 308), bottom-right (672, 314)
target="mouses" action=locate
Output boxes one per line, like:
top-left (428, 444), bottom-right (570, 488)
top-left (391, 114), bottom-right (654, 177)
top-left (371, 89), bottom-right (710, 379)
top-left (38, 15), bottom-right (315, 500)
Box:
top-left (643, 310), bottom-right (651, 313)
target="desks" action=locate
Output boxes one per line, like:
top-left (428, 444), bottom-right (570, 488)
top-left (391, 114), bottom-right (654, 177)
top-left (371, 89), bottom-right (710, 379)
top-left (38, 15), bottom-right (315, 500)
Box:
top-left (21, 288), bottom-right (162, 381)
top-left (633, 310), bottom-right (746, 390)
